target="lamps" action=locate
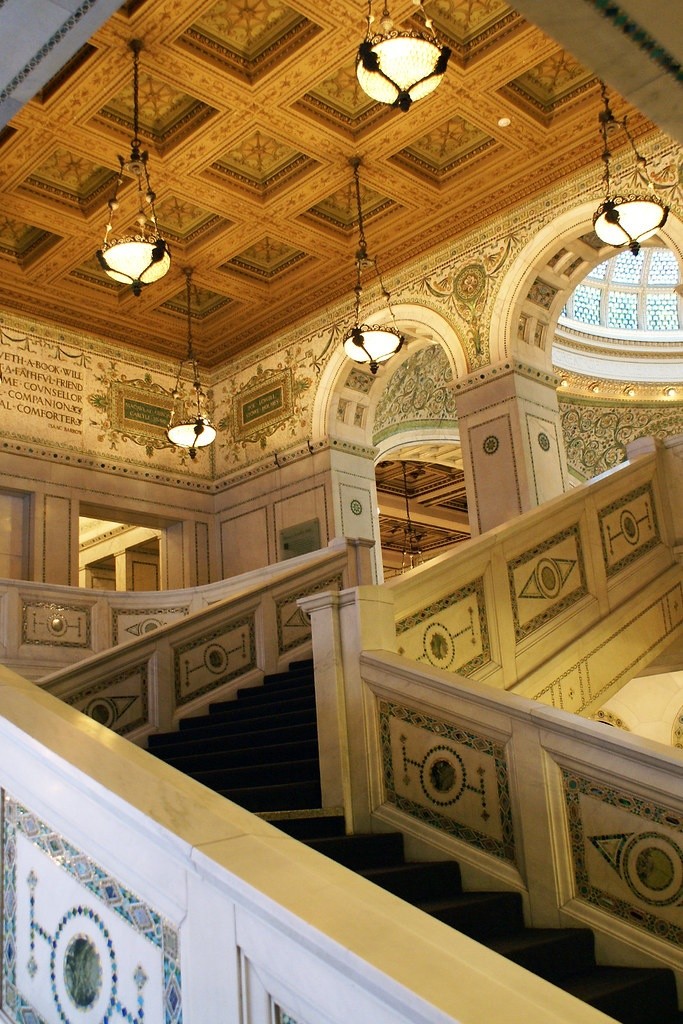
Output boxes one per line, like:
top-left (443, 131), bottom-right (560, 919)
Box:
top-left (342, 155), bottom-right (406, 374)
top-left (399, 460), bottom-right (430, 577)
top-left (166, 267), bottom-right (217, 458)
top-left (591, 83), bottom-right (669, 256)
top-left (356, 0), bottom-right (451, 112)
top-left (96, 43), bottom-right (172, 296)
